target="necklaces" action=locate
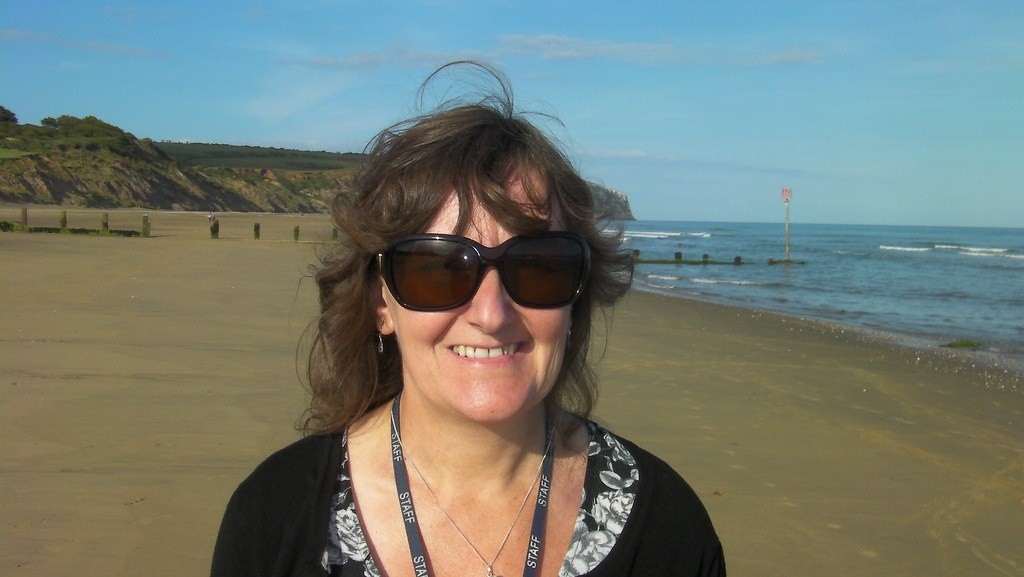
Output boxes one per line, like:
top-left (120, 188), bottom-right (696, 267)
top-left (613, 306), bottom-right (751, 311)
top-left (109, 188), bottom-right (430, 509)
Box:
top-left (388, 393), bottom-right (556, 577)
top-left (388, 407), bottom-right (555, 576)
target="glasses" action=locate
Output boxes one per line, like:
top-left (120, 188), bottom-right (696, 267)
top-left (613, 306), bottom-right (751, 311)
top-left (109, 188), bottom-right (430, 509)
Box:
top-left (373, 231), bottom-right (592, 313)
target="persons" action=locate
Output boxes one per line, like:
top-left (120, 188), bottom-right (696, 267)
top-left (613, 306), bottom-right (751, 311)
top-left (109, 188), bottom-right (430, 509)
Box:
top-left (207, 107), bottom-right (729, 577)
